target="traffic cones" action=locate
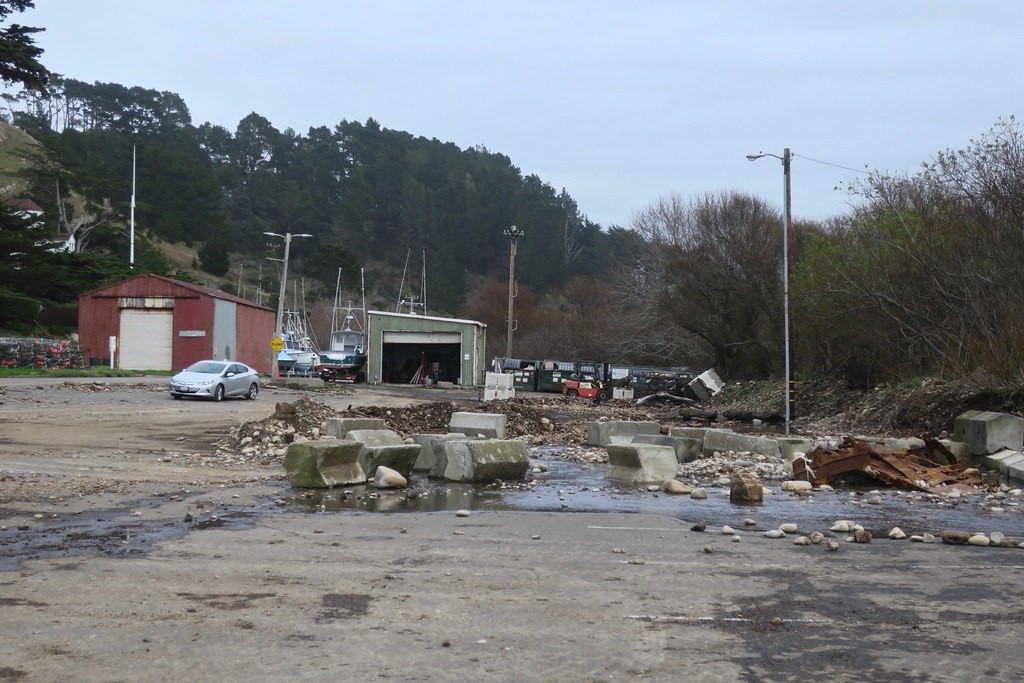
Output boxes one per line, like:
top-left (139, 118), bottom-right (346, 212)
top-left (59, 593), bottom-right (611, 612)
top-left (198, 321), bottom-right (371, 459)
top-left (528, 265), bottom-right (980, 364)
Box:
top-left (275, 367), bottom-right (280, 381)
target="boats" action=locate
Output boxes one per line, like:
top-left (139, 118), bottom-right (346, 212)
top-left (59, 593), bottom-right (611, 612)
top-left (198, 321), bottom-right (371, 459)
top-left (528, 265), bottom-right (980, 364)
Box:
top-left (277, 300), bottom-right (367, 375)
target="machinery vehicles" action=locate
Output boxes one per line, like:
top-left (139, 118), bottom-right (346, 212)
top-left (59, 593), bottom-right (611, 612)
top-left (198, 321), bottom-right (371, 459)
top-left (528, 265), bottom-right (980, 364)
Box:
top-left (560, 359), bottom-right (611, 401)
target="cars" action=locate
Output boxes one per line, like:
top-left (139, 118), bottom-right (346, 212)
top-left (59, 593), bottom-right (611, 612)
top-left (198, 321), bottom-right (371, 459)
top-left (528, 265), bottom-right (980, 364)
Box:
top-left (169, 359), bottom-right (261, 402)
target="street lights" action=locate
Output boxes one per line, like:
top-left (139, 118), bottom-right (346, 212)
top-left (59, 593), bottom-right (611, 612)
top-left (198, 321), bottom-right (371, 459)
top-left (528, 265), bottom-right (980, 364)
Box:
top-left (263, 231), bottom-right (314, 390)
top-left (502, 225), bottom-right (526, 359)
top-left (746, 148), bottom-right (794, 437)
